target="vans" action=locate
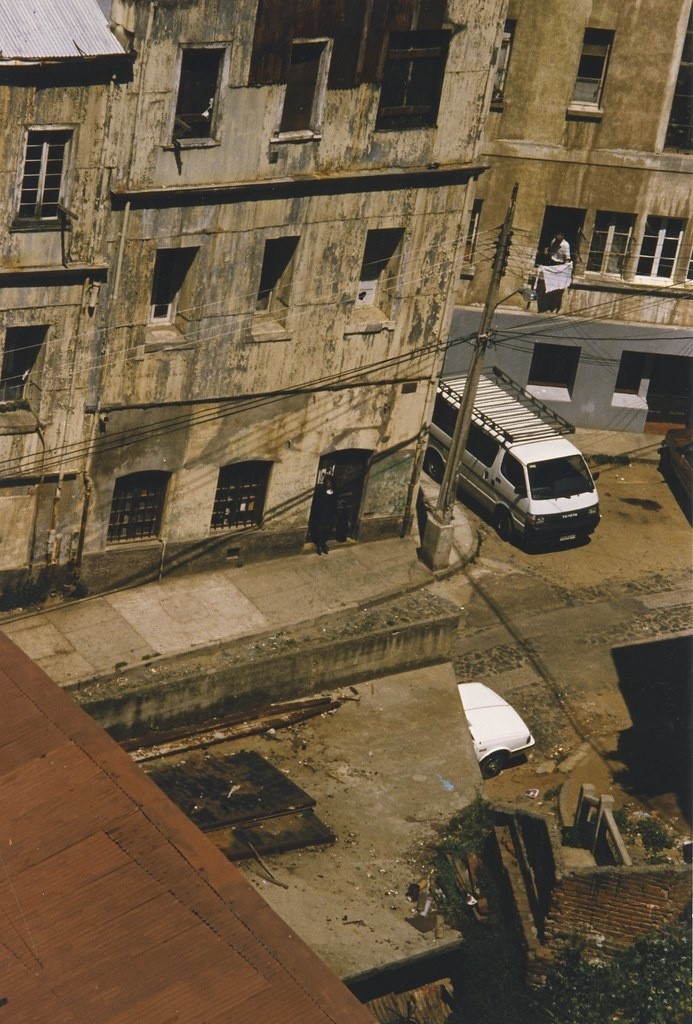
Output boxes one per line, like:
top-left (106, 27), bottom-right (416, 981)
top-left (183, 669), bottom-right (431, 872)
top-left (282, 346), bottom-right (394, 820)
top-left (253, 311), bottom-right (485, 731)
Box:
top-left (431, 362), bottom-right (602, 548)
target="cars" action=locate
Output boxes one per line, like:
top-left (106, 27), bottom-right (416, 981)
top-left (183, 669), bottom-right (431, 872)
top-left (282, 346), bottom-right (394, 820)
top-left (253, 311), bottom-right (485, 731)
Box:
top-left (457, 680), bottom-right (536, 776)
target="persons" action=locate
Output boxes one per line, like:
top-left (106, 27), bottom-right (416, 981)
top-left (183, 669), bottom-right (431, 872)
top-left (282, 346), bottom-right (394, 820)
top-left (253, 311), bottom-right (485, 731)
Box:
top-left (543, 231), bottom-right (571, 266)
top-left (308, 474), bottom-right (338, 556)
